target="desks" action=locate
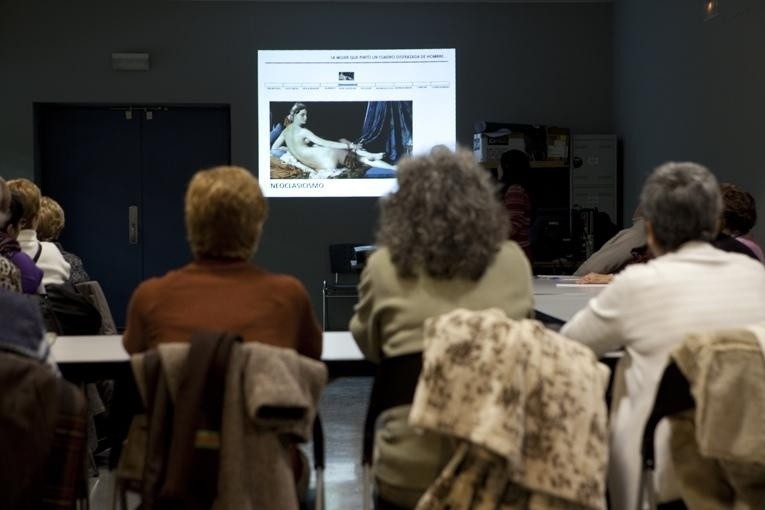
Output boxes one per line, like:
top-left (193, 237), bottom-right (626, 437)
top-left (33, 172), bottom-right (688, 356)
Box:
top-left (46, 328), bottom-right (367, 398)
top-left (528, 275), bottom-right (647, 356)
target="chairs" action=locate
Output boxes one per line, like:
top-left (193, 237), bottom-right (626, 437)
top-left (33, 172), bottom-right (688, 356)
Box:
top-left (321, 243), bottom-right (371, 332)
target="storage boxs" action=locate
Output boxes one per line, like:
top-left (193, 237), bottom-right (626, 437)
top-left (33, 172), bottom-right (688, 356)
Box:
top-left (473, 130), bottom-right (526, 163)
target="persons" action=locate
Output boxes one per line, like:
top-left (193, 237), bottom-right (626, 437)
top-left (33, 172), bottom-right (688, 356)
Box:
top-left (122, 162), bottom-right (324, 510)
top-left (558, 162), bottom-right (765, 510)
top-left (0, 288), bottom-right (86, 510)
top-left (271, 102), bottom-right (397, 172)
top-left (574, 179), bottom-right (764, 284)
top-left (571, 199), bottom-right (648, 277)
top-left (0, 174), bottom-right (91, 413)
top-left (346, 141), bottom-right (536, 509)
top-left (494, 148), bottom-right (534, 265)
